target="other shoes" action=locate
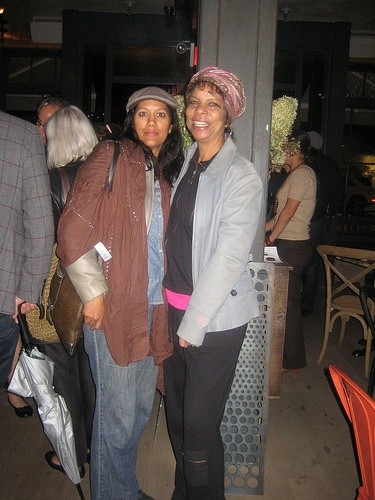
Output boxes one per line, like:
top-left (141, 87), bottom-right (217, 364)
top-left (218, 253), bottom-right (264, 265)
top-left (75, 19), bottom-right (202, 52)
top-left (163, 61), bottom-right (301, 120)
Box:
top-left (45, 451), bottom-right (85, 481)
top-left (85, 448), bottom-right (91, 465)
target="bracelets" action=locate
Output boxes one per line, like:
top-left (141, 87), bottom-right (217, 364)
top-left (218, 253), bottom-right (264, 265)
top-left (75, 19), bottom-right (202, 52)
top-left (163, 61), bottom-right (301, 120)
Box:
top-left (265, 237), bottom-right (273, 246)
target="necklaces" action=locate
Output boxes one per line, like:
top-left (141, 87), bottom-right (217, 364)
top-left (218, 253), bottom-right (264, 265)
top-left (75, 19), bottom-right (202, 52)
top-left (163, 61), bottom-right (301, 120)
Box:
top-left (272, 162), bottom-right (304, 214)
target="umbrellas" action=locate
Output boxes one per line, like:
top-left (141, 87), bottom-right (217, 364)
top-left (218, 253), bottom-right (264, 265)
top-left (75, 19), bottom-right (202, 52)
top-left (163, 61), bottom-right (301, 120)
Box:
top-left (7, 302), bottom-right (85, 500)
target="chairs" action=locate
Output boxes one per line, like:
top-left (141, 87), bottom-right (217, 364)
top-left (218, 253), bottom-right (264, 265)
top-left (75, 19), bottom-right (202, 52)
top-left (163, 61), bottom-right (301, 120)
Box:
top-left (358, 282), bottom-right (375, 398)
top-left (327, 363), bottom-right (375, 500)
top-left (316, 244), bottom-right (375, 380)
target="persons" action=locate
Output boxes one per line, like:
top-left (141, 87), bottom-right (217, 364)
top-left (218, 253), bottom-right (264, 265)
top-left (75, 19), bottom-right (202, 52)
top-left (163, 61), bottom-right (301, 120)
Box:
top-left (0, 98), bottom-right (110, 479)
top-left (162, 65), bottom-right (264, 500)
top-left (55, 87), bottom-right (186, 500)
top-left (265, 131), bottom-right (345, 371)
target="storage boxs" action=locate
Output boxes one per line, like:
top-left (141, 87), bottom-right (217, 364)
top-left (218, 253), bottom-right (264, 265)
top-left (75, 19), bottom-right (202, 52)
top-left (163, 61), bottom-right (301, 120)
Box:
top-left (30, 15), bottom-right (63, 45)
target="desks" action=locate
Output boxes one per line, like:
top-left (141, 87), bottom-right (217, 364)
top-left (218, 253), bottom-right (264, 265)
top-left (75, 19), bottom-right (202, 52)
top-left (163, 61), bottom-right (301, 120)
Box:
top-left (269, 257), bottom-right (294, 398)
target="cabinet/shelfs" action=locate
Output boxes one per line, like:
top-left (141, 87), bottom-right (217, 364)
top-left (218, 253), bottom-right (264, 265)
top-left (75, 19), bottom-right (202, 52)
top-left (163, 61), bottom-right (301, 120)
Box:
top-left (62, 8), bottom-right (352, 237)
top-left (0, 46), bottom-right (63, 113)
top-left (346, 56), bottom-right (375, 217)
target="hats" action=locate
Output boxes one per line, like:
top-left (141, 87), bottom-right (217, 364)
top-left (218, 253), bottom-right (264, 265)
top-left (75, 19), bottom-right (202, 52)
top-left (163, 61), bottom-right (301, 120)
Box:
top-left (184, 66), bottom-right (246, 121)
top-left (126, 86), bottom-right (179, 113)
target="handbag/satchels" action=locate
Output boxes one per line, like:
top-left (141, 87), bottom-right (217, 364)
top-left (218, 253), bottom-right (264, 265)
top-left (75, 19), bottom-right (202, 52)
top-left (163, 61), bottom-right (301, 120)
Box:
top-left (47, 259), bottom-right (85, 357)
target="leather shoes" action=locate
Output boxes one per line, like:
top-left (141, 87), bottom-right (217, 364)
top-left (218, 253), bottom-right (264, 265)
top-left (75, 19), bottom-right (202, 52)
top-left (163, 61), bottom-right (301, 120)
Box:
top-left (8, 394), bottom-right (33, 418)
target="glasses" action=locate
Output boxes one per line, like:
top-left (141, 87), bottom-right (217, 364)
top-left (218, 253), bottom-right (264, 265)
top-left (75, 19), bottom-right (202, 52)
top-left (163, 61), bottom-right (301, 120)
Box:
top-left (33, 114), bottom-right (47, 130)
